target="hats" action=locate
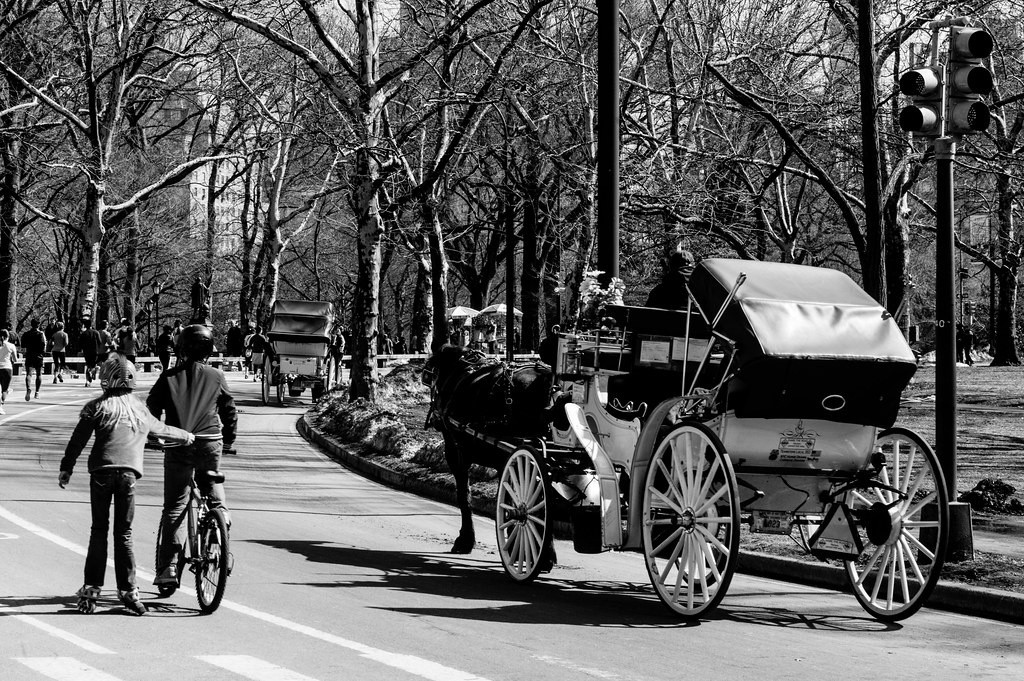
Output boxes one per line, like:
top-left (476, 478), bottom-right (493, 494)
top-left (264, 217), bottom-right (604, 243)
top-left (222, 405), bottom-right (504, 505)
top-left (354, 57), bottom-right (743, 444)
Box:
top-left (670, 251), bottom-right (696, 275)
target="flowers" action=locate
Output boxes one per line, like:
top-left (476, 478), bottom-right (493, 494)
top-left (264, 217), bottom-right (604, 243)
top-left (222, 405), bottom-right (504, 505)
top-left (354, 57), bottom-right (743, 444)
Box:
top-left (576, 268), bottom-right (626, 326)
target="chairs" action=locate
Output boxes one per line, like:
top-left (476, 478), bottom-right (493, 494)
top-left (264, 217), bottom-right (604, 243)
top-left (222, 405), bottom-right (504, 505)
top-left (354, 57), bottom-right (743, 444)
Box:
top-left (602, 302), bottom-right (710, 404)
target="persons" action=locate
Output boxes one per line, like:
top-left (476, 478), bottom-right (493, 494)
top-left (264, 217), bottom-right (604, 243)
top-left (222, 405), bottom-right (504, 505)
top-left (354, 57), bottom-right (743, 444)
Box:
top-left (156, 319), bottom-right (184, 372)
top-left (146, 324), bottom-right (238, 589)
top-left (58, 357), bottom-right (196, 615)
top-left (329, 327), bottom-right (346, 385)
top-left (628, 249), bottom-right (697, 368)
top-left (382, 331), bottom-right (427, 354)
top-left (956, 322), bottom-right (974, 366)
top-left (449, 322), bottom-right (497, 353)
top-left (0, 316), bottom-right (139, 414)
top-left (224, 320), bottom-right (269, 382)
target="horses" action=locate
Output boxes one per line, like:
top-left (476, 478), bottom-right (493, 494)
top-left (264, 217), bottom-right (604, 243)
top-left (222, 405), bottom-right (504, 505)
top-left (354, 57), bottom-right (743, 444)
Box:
top-left (419, 344), bottom-right (558, 571)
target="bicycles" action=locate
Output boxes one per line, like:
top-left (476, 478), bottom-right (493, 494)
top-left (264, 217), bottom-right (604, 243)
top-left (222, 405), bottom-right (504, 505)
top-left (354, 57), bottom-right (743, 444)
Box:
top-left (143, 442), bottom-right (237, 614)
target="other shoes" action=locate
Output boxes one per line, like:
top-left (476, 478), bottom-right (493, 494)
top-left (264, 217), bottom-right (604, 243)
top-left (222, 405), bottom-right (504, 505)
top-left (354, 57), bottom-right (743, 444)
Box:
top-left (152, 566), bottom-right (177, 583)
top-left (85, 383), bottom-right (90, 387)
top-left (0, 407), bottom-right (5, 415)
top-left (221, 511), bottom-right (232, 529)
top-left (89, 371), bottom-right (92, 382)
top-left (53, 377), bottom-right (57, 384)
top-left (58, 374), bottom-right (63, 382)
top-left (35, 392), bottom-right (39, 399)
top-left (25, 388), bottom-right (31, 401)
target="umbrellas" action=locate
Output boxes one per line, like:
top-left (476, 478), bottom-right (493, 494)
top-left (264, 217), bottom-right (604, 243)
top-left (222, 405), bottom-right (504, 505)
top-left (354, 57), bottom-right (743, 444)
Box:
top-left (480, 303), bottom-right (524, 336)
top-left (447, 306), bottom-right (479, 319)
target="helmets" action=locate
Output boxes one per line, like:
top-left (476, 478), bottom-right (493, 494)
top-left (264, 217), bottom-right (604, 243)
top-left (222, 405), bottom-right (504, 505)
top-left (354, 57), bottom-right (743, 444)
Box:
top-left (178, 325), bottom-right (214, 360)
top-left (99, 356), bottom-right (137, 389)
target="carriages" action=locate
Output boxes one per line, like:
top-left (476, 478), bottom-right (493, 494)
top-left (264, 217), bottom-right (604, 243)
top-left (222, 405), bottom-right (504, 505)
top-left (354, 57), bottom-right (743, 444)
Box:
top-left (262, 300), bottom-right (345, 405)
top-left (421, 256), bottom-right (951, 622)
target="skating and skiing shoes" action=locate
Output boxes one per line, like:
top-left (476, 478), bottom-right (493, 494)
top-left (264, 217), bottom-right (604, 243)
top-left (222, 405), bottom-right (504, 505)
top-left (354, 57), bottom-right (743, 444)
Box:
top-left (116, 589), bottom-right (146, 616)
top-left (75, 584), bottom-right (101, 614)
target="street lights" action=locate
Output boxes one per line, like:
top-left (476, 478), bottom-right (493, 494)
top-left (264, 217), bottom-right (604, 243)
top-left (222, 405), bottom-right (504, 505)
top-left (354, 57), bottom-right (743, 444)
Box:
top-left (151, 280), bottom-right (162, 357)
top-left (144, 298), bottom-right (154, 372)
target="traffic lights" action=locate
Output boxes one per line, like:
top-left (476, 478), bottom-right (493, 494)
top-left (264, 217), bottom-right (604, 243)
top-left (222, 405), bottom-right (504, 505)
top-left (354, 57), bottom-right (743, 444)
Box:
top-left (898, 67), bottom-right (944, 137)
top-left (948, 26), bottom-right (994, 135)
